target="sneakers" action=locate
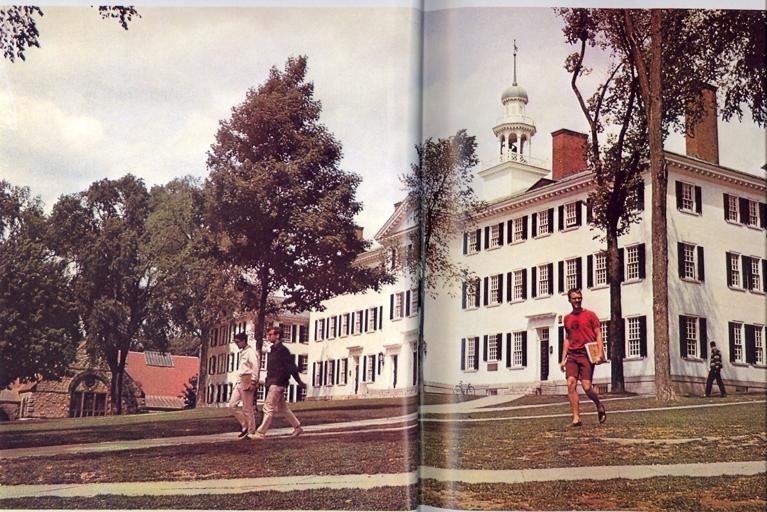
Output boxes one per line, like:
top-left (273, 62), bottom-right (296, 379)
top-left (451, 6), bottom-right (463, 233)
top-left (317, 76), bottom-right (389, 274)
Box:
top-left (291, 427), bottom-right (304, 436)
top-left (238, 428), bottom-right (266, 440)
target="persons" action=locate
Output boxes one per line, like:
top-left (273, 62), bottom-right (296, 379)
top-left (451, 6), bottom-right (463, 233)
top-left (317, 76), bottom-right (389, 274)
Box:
top-left (246, 326), bottom-right (304, 437)
top-left (701, 341), bottom-right (727, 398)
top-left (559, 287), bottom-right (607, 428)
top-left (228, 333), bottom-right (261, 440)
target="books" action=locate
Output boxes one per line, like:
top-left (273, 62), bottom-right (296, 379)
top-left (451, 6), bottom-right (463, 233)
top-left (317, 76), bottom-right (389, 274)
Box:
top-left (584, 342), bottom-right (609, 363)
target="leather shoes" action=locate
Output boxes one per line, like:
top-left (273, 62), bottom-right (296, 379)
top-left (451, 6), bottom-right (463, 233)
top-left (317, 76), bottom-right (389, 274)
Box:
top-left (566, 419), bottom-right (583, 427)
top-left (597, 405), bottom-right (606, 423)
